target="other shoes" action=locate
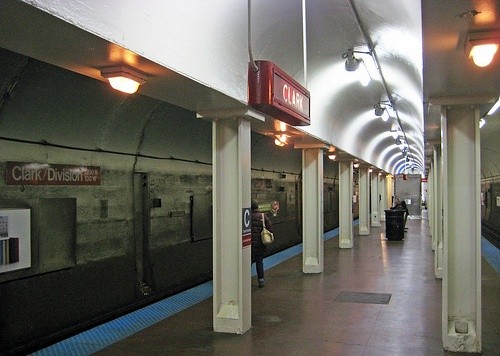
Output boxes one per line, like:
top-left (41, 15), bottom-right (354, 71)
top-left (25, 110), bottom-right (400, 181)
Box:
top-left (259, 281), bottom-right (264, 287)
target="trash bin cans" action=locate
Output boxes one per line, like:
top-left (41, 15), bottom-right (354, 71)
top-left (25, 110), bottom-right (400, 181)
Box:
top-left (385, 209), bottom-right (406, 241)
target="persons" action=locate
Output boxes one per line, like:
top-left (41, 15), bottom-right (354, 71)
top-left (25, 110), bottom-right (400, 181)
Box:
top-left (251, 199), bottom-right (273, 286)
top-left (390, 198), bottom-right (401, 210)
top-left (401, 200), bottom-right (409, 229)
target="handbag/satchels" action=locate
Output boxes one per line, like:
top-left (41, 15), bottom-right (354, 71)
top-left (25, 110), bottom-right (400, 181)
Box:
top-left (261, 213), bottom-right (275, 244)
